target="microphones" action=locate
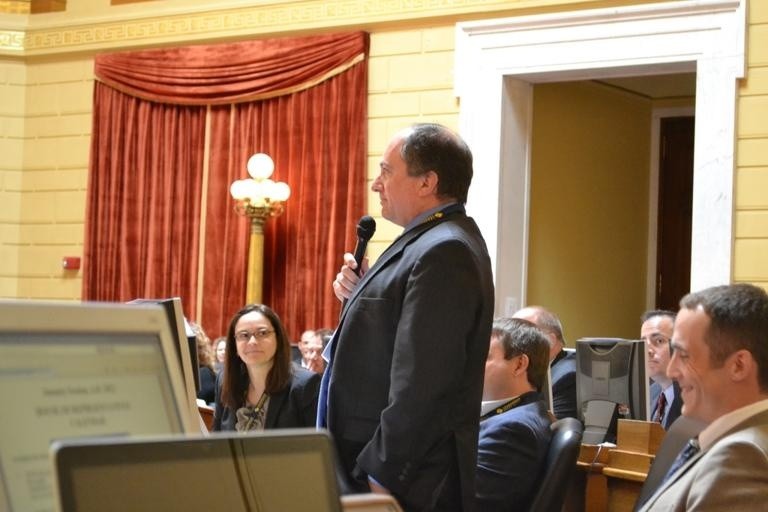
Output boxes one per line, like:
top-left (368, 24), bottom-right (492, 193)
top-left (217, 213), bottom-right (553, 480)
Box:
top-left (341, 215), bottom-right (376, 313)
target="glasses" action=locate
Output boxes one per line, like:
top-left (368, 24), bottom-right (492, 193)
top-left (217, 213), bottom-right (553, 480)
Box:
top-left (233, 329), bottom-right (275, 341)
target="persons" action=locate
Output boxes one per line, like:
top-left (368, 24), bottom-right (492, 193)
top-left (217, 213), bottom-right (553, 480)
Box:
top-left (307, 123), bottom-right (495, 512)
top-left (474, 317), bottom-right (550, 511)
top-left (185, 303), bottom-right (334, 433)
top-left (635, 284), bottom-right (768, 511)
top-left (639, 312), bottom-right (683, 424)
top-left (508, 307), bottom-right (577, 422)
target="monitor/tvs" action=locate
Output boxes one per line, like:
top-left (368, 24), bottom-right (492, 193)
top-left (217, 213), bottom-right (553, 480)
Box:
top-left (125, 297), bottom-right (202, 434)
top-left (0, 298), bottom-right (194, 512)
top-left (575, 337), bottom-right (651, 445)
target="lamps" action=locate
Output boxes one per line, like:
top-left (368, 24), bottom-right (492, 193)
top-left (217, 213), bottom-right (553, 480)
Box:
top-left (229, 150), bottom-right (292, 311)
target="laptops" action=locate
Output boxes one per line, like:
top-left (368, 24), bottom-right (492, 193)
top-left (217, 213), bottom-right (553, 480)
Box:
top-left (48, 427), bottom-right (344, 512)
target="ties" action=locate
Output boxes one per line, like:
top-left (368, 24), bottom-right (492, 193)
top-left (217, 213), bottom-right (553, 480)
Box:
top-left (638, 438), bottom-right (699, 509)
top-left (653, 392), bottom-right (665, 423)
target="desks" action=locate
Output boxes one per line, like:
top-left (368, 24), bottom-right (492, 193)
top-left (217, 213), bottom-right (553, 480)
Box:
top-left (576, 461), bottom-right (647, 511)
top-left (197, 403), bottom-right (213, 433)
